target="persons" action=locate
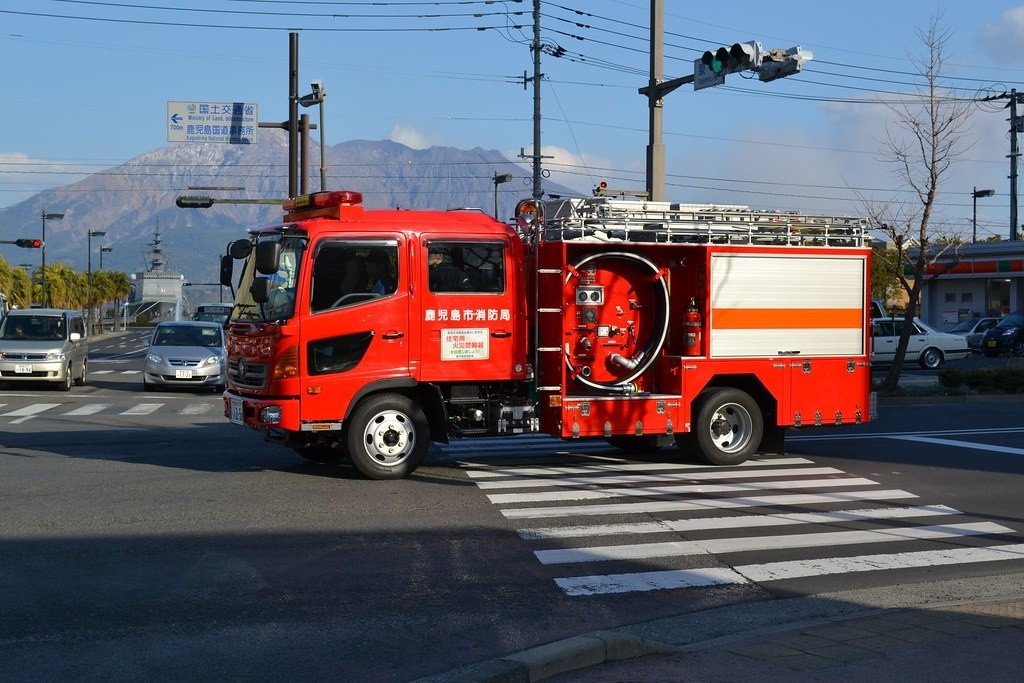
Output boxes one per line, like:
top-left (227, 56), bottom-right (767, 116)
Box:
top-left (44, 325), bottom-right (63, 338)
top-left (429, 246), bottom-right (472, 292)
top-left (364, 248), bottom-right (396, 299)
top-left (10, 324), bottom-right (24, 336)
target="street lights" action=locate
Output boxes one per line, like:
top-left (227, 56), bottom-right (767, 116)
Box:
top-left (98, 246), bottom-right (113, 334)
top-left (310, 79), bottom-right (329, 192)
top-left (39, 209), bottom-right (66, 309)
top-left (86, 229), bottom-right (106, 336)
top-left (972, 185), bottom-right (995, 244)
top-left (284, 32), bottom-right (323, 199)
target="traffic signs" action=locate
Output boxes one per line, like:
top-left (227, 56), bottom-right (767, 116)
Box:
top-left (166, 100), bottom-right (259, 144)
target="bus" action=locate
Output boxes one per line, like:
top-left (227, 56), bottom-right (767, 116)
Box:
top-left (0, 292), bottom-right (10, 321)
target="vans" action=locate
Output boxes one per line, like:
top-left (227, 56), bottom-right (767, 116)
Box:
top-left (0, 307), bottom-right (88, 391)
top-left (871, 298), bottom-right (888, 319)
top-left (192, 301), bottom-right (235, 336)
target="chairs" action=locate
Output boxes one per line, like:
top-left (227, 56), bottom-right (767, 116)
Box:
top-left (157, 333), bottom-right (178, 344)
top-left (472, 263), bottom-right (503, 292)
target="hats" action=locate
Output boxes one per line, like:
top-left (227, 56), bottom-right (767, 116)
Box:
top-left (360, 249), bottom-right (390, 263)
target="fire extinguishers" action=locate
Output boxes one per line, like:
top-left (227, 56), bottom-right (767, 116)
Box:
top-left (681, 297), bottom-right (703, 357)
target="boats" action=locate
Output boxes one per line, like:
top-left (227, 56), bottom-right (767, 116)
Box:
top-left (120, 213), bottom-right (186, 324)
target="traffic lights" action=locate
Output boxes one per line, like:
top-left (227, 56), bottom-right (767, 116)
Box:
top-left (183, 282), bottom-right (192, 286)
top-left (15, 238), bottom-right (45, 248)
top-left (701, 39), bottom-right (758, 76)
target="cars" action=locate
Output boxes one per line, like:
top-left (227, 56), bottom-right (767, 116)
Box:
top-left (141, 320), bottom-right (228, 393)
top-left (871, 317), bottom-right (973, 370)
top-left (947, 316), bottom-right (1004, 349)
top-left (979, 309), bottom-right (1024, 357)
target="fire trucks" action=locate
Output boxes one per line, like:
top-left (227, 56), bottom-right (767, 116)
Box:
top-left (218, 183), bottom-right (889, 482)
